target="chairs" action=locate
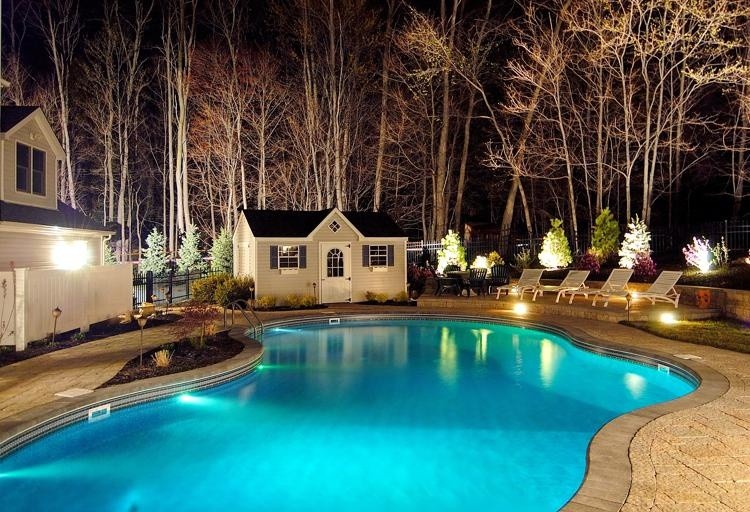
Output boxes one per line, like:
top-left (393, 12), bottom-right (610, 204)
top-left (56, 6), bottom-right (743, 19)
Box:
top-left (429, 263), bottom-right (683, 311)
